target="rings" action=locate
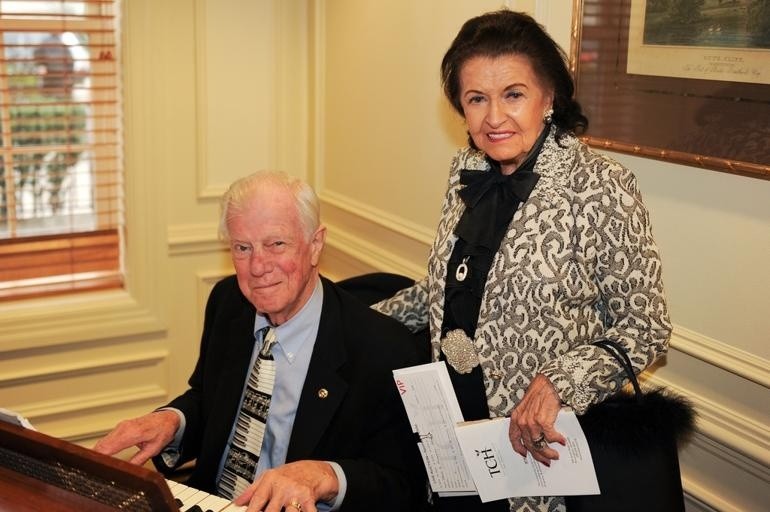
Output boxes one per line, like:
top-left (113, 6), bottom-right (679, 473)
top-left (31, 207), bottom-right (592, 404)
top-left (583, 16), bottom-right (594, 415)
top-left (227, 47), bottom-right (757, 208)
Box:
top-left (288, 501), bottom-right (302, 512)
top-left (532, 434), bottom-right (547, 449)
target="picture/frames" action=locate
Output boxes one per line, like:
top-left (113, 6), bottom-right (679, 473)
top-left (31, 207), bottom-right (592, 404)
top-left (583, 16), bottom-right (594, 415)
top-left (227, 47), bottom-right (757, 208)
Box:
top-left (567, 0), bottom-right (770, 181)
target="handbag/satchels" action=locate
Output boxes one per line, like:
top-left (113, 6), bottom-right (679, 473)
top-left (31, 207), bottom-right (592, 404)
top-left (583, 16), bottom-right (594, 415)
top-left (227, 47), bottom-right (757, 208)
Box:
top-left (564, 387), bottom-right (693, 510)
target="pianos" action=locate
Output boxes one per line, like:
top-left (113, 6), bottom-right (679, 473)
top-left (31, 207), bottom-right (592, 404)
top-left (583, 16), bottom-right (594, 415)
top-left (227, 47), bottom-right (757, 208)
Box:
top-left (0, 419), bottom-right (251, 512)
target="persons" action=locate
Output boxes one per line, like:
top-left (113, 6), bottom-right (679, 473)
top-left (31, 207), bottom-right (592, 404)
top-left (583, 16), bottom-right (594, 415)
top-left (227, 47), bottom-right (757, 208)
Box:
top-left (368, 9), bottom-right (675, 512)
top-left (32, 32), bottom-right (75, 101)
top-left (92, 169), bottom-right (427, 512)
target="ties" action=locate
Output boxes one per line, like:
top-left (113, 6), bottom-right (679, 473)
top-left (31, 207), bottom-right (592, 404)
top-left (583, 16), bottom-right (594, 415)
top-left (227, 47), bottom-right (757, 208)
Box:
top-left (214, 328), bottom-right (278, 507)
